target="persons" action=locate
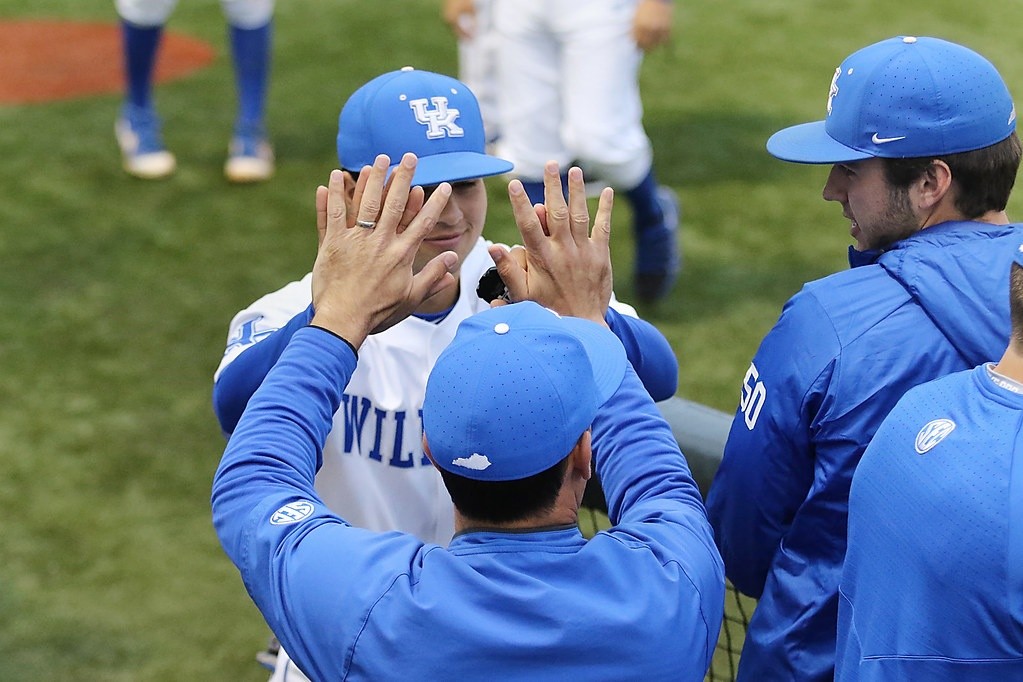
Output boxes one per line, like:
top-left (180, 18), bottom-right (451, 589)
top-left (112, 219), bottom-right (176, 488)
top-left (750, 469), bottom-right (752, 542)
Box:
top-left (113, 0), bottom-right (278, 180)
top-left (834, 245), bottom-right (1023, 682)
top-left (215, 67), bottom-right (678, 682)
top-left (442, 1), bottom-right (677, 299)
top-left (211, 154), bottom-right (725, 682)
top-left (706, 36), bottom-right (1023, 682)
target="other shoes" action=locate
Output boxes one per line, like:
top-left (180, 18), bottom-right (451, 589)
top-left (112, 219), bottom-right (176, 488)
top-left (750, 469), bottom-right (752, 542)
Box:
top-left (223, 136), bottom-right (280, 183)
top-left (116, 120), bottom-right (177, 180)
top-left (624, 191), bottom-right (679, 304)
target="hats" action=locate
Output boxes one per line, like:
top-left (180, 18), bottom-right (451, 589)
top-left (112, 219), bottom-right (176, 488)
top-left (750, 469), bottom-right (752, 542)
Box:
top-left (766, 36), bottom-right (1015, 165)
top-left (421, 299), bottom-right (627, 482)
top-left (337, 65), bottom-right (515, 189)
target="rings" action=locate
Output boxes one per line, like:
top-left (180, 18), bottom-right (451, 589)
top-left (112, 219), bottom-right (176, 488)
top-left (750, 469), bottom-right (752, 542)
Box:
top-left (355, 221), bottom-right (376, 228)
